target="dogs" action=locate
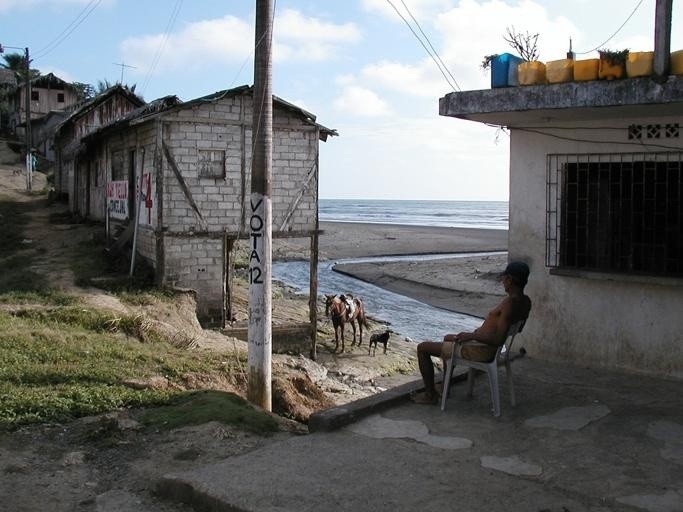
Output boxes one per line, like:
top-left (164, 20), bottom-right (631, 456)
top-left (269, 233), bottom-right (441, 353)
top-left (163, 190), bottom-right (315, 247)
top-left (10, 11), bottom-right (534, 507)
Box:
top-left (368, 329), bottom-right (394, 356)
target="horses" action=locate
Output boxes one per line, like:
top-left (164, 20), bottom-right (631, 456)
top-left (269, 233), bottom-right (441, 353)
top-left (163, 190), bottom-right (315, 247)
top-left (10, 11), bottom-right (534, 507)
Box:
top-left (323, 292), bottom-right (371, 354)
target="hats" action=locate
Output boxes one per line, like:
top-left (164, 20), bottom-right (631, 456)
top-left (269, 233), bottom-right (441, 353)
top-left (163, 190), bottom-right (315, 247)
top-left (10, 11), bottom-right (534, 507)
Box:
top-left (498, 261), bottom-right (531, 279)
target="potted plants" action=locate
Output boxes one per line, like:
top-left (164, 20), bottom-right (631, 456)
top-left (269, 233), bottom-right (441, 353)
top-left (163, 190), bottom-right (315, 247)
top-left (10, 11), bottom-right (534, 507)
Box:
top-left (502, 24), bottom-right (546, 86)
top-left (478, 52), bottom-right (526, 89)
top-left (596, 48), bottom-right (629, 81)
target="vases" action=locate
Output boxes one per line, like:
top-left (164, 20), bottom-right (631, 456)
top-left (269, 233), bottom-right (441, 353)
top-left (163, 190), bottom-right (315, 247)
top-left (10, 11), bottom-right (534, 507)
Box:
top-left (625, 52), bottom-right (655, 80)
top-left (573, 59), bottom-right (599, 82)
top-left (669, 49), bottom-right (683, 75)
top-left (545, 59), bottom-right (572, 83)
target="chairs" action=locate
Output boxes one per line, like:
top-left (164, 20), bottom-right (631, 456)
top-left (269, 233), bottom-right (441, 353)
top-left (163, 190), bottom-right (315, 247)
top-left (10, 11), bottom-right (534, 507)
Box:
top-left (440, 313), bottom-right (525, 417)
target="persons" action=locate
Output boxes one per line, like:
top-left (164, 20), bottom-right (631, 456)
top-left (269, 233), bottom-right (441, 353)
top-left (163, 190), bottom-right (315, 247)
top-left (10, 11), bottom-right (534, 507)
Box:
top-left (410, 262), bottom-right (531, 405)
